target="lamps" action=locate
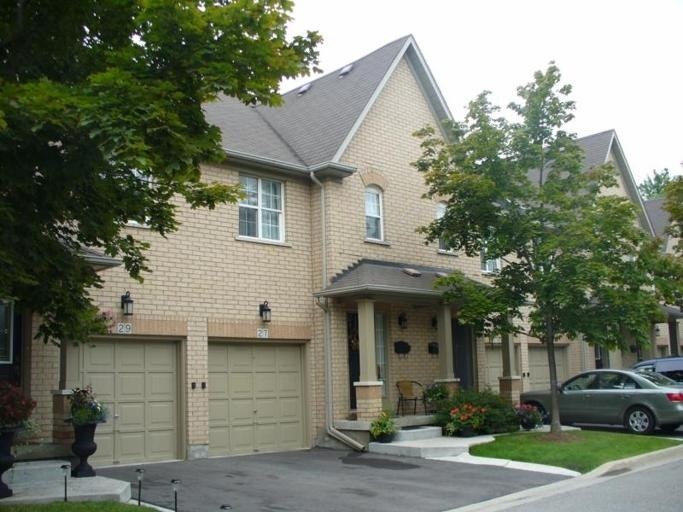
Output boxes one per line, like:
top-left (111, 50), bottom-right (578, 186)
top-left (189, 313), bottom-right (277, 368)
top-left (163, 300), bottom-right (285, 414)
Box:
top-left (656, 325), bottom-right (660, 336)
top-left (121, 291), bottom-right (134, 314)
top-left (432, 315), bottom-right (438, 329)
top-left (399, 316), bottom-right (407, 329)
top-left (260, 301), bottom-right (272, 322)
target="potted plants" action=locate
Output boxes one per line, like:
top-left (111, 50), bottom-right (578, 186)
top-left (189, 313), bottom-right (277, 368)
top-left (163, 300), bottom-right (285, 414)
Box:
top-left (426, 385), bottom-right (450, 414)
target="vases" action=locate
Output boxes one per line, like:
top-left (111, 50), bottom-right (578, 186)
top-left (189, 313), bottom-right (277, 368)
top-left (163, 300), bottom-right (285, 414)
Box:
top-left (522, 420), bottom-right (536, 429)
top-left (378, 433), bottom-right (391, 442)
top-left (65, 419), bottom-right (104, 477)
top-left (460, 424), bottom-right (479, 436)
top-left (0, 427), bottom-right (23, 498)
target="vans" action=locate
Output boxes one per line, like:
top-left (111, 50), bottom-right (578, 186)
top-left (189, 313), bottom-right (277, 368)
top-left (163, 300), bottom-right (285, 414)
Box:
top-left (613, 358), bottom-right (682, 390)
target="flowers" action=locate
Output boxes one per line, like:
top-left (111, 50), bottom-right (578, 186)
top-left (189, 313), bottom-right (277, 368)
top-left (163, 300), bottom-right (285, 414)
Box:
top-left (445, 403), bottom-right (488, 435)
top-left (370, 411), bottom-right (397, 439)
top-left (0, 379), bottom-right (39, 432)
top-left (516, 404), bottom-right (550, 431)
top-left (68, 383), bottom-right (110, 427)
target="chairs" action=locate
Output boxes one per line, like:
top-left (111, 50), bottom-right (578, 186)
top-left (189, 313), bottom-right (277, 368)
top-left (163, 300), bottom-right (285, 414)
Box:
top-left (397, 380), bottom-right (427, 416)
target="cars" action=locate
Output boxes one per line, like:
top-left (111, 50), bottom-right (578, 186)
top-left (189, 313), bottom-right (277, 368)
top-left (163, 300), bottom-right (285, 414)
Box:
top-left (520, 368), bottom-right (683, 435)
